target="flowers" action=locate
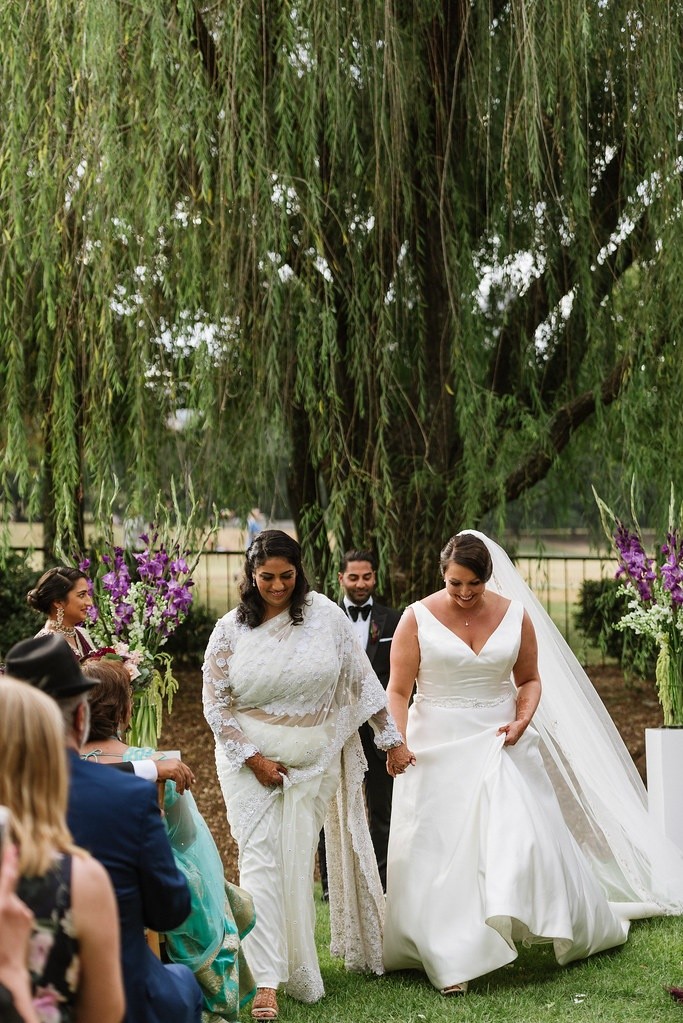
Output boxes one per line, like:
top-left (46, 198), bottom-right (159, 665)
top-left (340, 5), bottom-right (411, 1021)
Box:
top-left (73, 471), bottom-right (220, 749)
top-left (587, 470), bottom-right (682, 728)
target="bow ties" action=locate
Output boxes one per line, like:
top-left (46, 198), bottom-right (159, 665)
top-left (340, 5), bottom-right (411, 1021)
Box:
top-left (348, 604), bottom-right (371, 622)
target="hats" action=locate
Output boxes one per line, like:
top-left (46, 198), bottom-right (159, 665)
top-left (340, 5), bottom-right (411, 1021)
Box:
top-left (6, 633), bottom-right (100, 695)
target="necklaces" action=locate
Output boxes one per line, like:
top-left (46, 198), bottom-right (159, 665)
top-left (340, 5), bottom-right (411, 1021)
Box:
top-left (46, 618), bottom-right (76, 638)
top-left (447, 596), bottom-right (485, 626)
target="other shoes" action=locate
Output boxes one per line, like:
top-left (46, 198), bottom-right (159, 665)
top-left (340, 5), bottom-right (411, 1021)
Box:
top-left (252, 987), bottom-right (279, 1020)
top-left (440, 980), bottom-right (470, 994)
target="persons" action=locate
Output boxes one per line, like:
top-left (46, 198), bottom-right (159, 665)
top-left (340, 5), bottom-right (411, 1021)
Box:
top-left (384, 535), bottom-right (544, 997)
top-left (199, 530), bottom-right (416, 1022)
top-left (27, 567), bottom-right (99, 661)
top-left (316, 550), bottom-right (415, 903)
top-left (0, 631), bottom-right (257, 1022)
